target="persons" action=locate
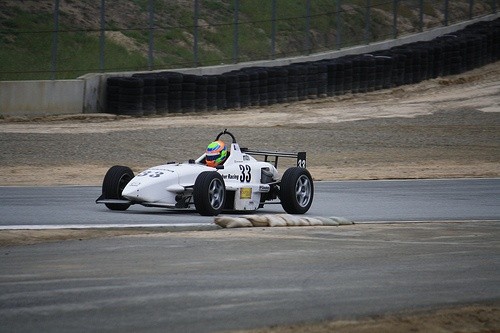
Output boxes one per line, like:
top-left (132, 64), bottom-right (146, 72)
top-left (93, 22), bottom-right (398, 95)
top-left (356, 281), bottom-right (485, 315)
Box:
top-left (204, 140), bottom-right (228, 168)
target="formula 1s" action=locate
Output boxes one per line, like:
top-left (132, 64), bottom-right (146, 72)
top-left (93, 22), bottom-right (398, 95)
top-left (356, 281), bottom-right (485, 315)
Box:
top-left (95, 126), bottom-right (314, 216)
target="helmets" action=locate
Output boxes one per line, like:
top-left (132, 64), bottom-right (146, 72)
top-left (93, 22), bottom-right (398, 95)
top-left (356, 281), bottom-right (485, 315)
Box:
top-left (206, 140), bottom-right (227, 167)
